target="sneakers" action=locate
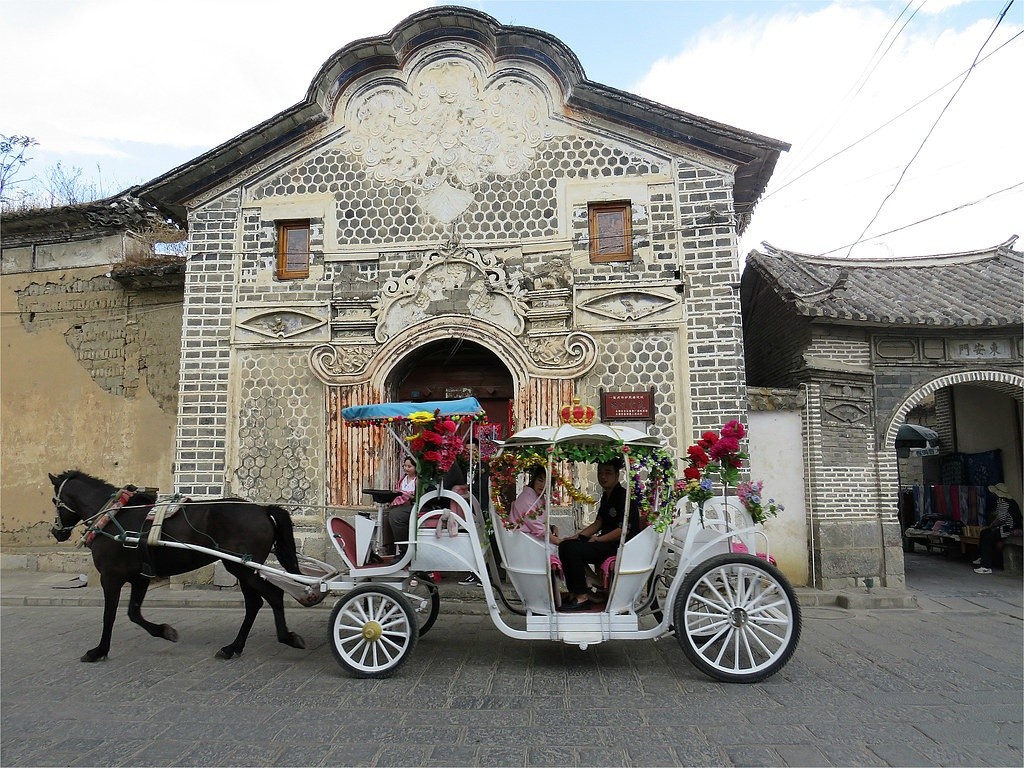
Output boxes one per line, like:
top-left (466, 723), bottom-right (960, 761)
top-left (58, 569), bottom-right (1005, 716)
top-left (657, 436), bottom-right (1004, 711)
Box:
top-left (458, 575), bottom-right (483, 587)
top-left (973, 558), bottom-right (981, 565)
top-left (974, 567), bottom-right (992, 574)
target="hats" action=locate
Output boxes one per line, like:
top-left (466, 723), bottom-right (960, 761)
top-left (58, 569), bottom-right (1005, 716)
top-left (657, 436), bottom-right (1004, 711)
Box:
top-left (988, 483), bottom-right (1013, 499)
top-left (922, 519), bottom-right (945, 530)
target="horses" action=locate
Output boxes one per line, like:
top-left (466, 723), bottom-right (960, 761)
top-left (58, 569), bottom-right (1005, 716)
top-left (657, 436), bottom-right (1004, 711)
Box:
top-left (48, 469), bottom-right (312, 662)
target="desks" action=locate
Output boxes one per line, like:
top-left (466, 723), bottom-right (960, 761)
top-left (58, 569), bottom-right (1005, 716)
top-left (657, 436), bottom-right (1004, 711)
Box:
top-left (908, 528), bottom-right (981, 558)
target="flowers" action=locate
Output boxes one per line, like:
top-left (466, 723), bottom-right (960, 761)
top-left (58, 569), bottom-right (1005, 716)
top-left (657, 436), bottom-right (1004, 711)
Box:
top-left (408, 410), bottom-right (786, 533)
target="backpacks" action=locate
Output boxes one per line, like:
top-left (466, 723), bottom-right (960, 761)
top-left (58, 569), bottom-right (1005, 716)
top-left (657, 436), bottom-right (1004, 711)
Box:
top-left (1002, 498), bottom-right (1023, 529)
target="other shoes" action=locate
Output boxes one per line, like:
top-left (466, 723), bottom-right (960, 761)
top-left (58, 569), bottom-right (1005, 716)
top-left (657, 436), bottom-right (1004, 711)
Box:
top-left (390, 555), bottom-right (404, 565)
top-left (588, 594), bottom-right (602, 603)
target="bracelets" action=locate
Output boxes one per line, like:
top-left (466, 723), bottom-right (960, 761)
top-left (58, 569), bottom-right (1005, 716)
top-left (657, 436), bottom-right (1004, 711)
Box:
top-left (551, 525), bottom-right (555, 529)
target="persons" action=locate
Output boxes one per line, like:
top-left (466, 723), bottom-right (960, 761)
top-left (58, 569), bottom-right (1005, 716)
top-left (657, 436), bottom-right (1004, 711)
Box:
top-left (972, 483), bottom-right (1013, 573)
top-left (509, 458), bottom-right (640, 612)
top-left (383, 437), bottom-right (490, 587)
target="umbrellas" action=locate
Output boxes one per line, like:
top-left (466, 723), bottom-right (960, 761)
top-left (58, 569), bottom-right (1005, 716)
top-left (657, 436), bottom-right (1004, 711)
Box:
top-left (895, 424), bottom-right (940, 536)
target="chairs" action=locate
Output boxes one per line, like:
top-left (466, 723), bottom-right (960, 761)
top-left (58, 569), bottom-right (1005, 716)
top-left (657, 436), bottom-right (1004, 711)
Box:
top-left (420, 484), bottom-right (467, 527)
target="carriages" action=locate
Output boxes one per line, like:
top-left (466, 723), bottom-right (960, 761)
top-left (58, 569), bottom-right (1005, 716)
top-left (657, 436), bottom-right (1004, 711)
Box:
top-left (50, 398), bottom-right (806, 684)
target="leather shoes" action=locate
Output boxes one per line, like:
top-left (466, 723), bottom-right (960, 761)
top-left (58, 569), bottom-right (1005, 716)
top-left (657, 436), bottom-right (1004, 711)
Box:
top-left (555, 598), bottom-right (591, 611)
